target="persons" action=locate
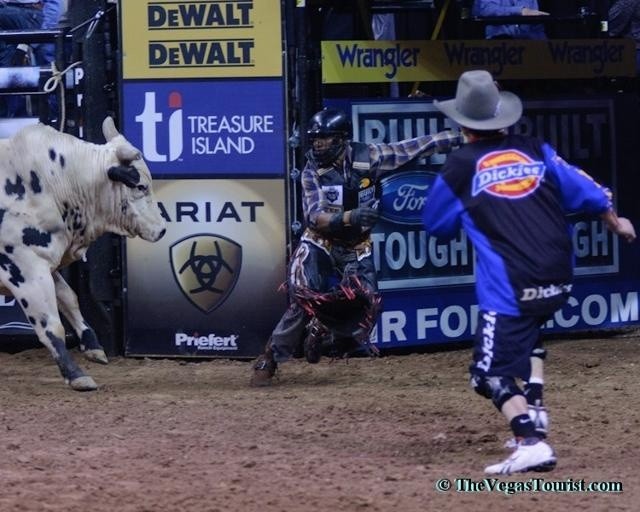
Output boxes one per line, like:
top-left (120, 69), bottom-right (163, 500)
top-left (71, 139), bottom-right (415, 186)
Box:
top-left (608, 0), bottom-right (640, 83)
top-left (471, 0), bottom-right (548, 40)
top-left (0, 0), bottom-right (67, 133)
top-left (250, 108), bottom-right (465, 387)
top-left (422, 70), bottom-right (636, 476)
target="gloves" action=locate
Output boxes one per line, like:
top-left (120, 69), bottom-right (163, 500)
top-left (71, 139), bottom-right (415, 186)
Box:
top-left (348, 205), bottom-right (382, 230)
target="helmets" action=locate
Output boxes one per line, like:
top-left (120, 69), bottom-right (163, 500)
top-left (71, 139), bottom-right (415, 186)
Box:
top-left (304, 106), bottom-right (355, 166)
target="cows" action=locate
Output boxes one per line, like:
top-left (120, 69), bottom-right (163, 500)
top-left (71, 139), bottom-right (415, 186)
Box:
top-left (0, 116), bottom-right (167, 392)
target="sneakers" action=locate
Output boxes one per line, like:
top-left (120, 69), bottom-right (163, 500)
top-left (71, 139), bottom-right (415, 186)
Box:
top-left (303, 316), bottom-right (327, 366)
top-left (502, 404), bottom-right (551, 450)
top-left (483, 437), bottom-right (559, 478)
top-left (250, 350), bottom-right (278, 388)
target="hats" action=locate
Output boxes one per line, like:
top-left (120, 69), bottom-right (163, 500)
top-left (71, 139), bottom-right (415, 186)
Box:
top-left (431, 67), bottom-right (526, 134)
